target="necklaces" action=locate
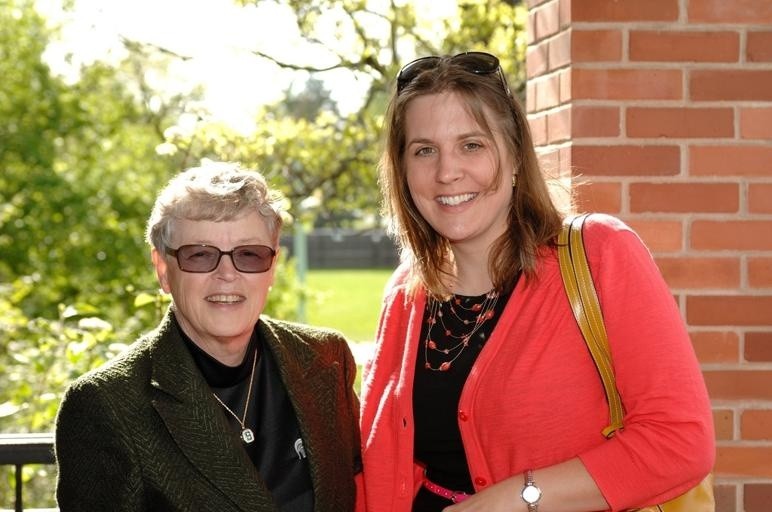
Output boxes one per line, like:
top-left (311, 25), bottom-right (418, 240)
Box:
top-left (213, 349), bottom-right (258, 445)
top-left (425, 287), bottom-right (500, 371)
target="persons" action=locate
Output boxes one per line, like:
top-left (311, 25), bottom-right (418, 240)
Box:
top-left (355, 53), bottom-right (718, 512)
top-left (55, 160), bottom-right (363, 512)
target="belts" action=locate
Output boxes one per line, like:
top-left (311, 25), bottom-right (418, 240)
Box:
top-left (421, 469), bottom-right (471, 504)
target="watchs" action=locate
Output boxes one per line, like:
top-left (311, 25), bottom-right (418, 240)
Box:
top-left (520, 469), bottom-right (542, 512)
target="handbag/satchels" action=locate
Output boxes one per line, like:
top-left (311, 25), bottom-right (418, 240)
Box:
top-left (635, 474), bottom-right (715, 512)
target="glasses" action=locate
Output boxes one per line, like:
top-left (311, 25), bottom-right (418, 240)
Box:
top-left (166, 244), bottom-right (278, 273)
top-left (398, 52), bottom-right (522, 149)
